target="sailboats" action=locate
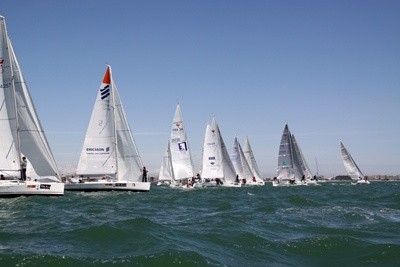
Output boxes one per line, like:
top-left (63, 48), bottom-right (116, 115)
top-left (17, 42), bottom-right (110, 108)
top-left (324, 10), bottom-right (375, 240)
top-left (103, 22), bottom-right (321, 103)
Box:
top-left (63, 65), bottom-right (151, 192)
top-left (339, 141), bottom-right (371, 186)
top-left (272, 124), bottom-right (308, 186)
top-left (168, 104), bottom-right (197, 189)
top-left (0, 16), bottom-right (65, 196)
top-left (243, 136), bottom-right (265, 185)
top-left (291, 134), bottom-right (317, 186)
top-left (231, 137), bottom-right (260, 186)
top-left (158, 138), bottom-right (172, 186)
top-left (200, 115), bottom-right (242, 188)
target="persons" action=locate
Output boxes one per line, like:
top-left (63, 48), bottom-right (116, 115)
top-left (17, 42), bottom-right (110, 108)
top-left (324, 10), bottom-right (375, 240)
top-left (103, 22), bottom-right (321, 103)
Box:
top-left (187, 174), bottom-right (318, 187)
top-left (0, 175), bottom-right (5, 180)
top-left (19, 157), bottom-right (27, 181)
top-left (142, 167), bottom-right (148, 182)
